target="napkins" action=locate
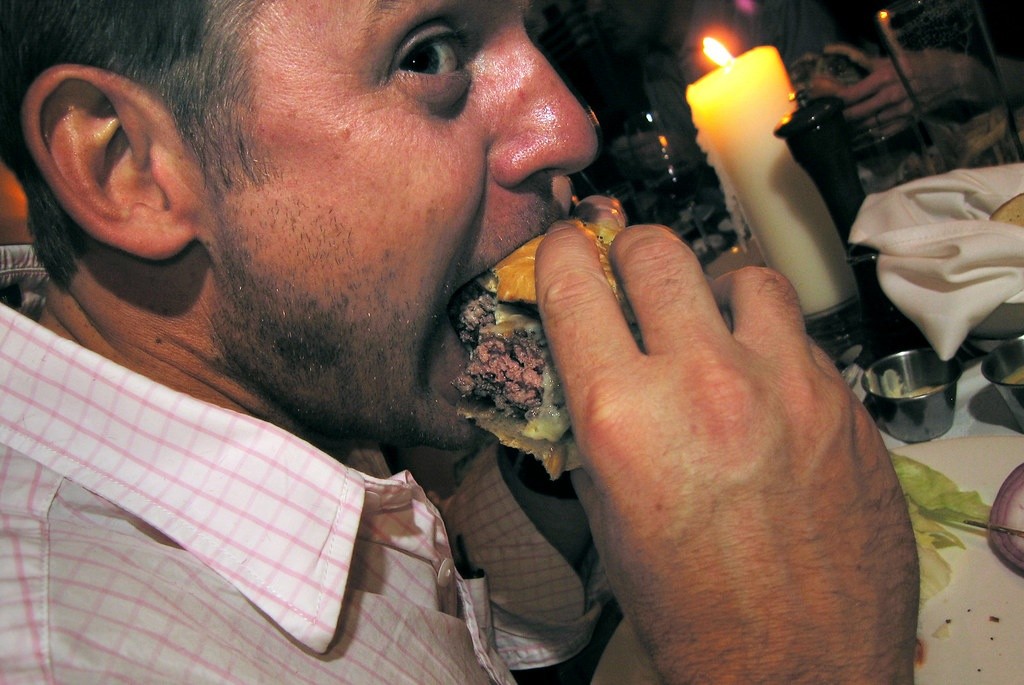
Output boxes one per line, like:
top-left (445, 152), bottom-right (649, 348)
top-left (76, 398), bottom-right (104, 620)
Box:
top-left (848, 160), bottom-right (1024, 361)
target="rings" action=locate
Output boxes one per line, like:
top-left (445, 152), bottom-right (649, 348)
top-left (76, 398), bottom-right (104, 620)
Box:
top-left (874, 114), bottom-right (883, 126)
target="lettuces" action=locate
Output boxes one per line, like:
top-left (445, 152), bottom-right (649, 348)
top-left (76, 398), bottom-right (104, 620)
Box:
top-left (887, 449), bottom-right (995, 614)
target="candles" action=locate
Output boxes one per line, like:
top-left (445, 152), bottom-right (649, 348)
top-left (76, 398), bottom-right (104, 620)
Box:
top-left (682, 28), bottom-right (870, 318)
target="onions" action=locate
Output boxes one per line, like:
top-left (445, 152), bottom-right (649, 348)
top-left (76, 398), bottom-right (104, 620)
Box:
top-left (988, 462), bottom-right (1024, 573)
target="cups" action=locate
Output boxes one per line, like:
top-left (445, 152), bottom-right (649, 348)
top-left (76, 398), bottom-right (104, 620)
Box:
top-left (850, 115), bottom-right (937, 196)
top-left (919, 77), bottom-right (1023, 173)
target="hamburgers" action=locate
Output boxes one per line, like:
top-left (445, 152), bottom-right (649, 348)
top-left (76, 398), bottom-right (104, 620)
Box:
top-left (456, 221), bottom-right (641, 482)
top-left (788, 51), bottom-right (817, 90)
top-left (806, 42), bottom-right (873, 99)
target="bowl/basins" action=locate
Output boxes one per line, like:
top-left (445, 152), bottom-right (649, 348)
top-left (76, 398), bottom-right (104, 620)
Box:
top-left (860, 347), bottom-right (963, 443)
top-left (981, 332), bottom-right (1024, 433)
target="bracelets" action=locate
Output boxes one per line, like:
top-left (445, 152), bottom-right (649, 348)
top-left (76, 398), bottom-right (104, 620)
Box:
top-left (521, 456), bottom-right (577, 500)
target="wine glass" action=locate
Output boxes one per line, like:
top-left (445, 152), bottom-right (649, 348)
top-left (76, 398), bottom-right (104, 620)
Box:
top-left (624, 104), bottom-right (732, 259)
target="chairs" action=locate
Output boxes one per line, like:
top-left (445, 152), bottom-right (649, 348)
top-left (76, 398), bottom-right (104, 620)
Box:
top-left (878, 0), bottom-right (1024, 170)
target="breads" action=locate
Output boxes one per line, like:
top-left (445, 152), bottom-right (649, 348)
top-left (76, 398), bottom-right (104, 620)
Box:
top-left (989, 192), bottom-right (1024, 229)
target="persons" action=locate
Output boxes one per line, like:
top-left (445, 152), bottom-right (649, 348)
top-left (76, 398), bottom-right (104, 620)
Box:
top-left (675, 0), bottom-right (1024, 150)
top-left (1, 0), bottom-right (918, 685)
